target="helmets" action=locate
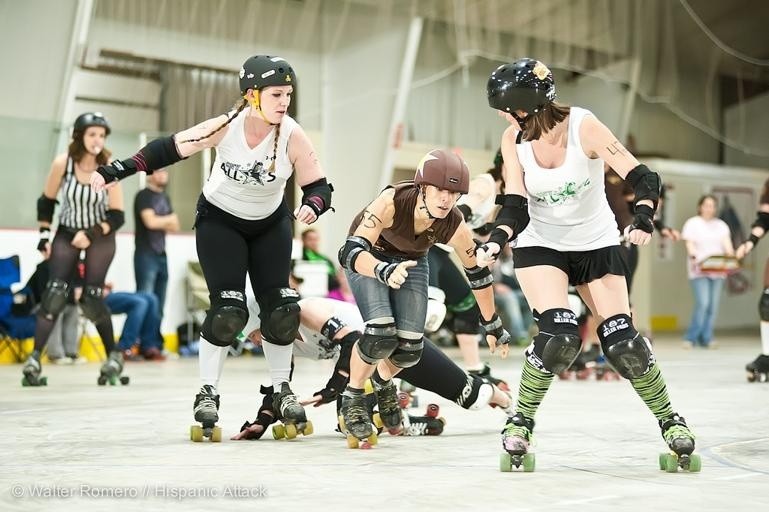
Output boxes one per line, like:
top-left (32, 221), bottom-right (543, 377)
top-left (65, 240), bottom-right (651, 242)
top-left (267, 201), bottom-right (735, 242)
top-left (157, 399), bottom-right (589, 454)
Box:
top-left (487, 58), bottom-right (556, 114)
top-left (415, 149), bottom-right (468, 194)
top-left (74, 112), bottom-right (110, 132)
top-left (239, 56), bottom-right (296, 96)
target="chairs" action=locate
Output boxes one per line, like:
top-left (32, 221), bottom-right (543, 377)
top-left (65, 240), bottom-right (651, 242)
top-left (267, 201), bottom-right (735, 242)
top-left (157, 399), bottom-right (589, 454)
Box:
top-left (0, 255), bottom-right (37, 363)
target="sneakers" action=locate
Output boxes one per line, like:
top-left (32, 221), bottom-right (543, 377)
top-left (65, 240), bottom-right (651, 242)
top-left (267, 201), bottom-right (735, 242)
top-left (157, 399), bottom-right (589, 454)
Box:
top-left (75, 357), bottom-right (86, 364)
top-left (142, 348), bottom-right (165, 360)
top-left (126, 350), bottom-right (144, 361)
top-left (55, 358), bottom-right (73, 366)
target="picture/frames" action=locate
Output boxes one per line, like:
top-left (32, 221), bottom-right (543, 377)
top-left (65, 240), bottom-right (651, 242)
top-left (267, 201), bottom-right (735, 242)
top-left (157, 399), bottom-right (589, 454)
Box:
top-left (709, 185), bottom-right (756, 297)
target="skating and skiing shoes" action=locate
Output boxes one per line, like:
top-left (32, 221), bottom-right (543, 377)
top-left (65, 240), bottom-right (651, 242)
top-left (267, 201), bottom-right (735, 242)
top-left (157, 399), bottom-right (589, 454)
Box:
top-left (659, 413), bottom-right (701, 473)
top-left (98, 353), bottom-right (129, 385)
top-left (746, 354), bottom-right (769, 381)
top-left (23, 359), bottom-right (47, 385)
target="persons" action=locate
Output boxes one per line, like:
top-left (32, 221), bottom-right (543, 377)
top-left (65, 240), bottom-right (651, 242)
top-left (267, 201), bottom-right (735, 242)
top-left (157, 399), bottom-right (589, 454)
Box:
top-left (330, 270), bottom-right (357, 305)
top-left (230, 289), bottom-right (513, 441)
top-left (88, 54), bottom-right (335, 425)
top-left (132, 165), bottom-right (177, 358)
top-left (302, 227), bottom-right (340, 289)
top-left (567, 155), bottom-right (680, 369)
top-left (681, 194), bottom-right (733, 354)
top-left (35, 237), bottom-right (167, 359)
top-left (465, 56), bottom-right (696, 457)
top-left (735, 177), bottom-right (769, 373)
top-left (21, 110), bottom-right (124, 377)
top-left (398, 148), bottom-right (538, 396)
top-left (336, 147), bottom-right (511, 439)
